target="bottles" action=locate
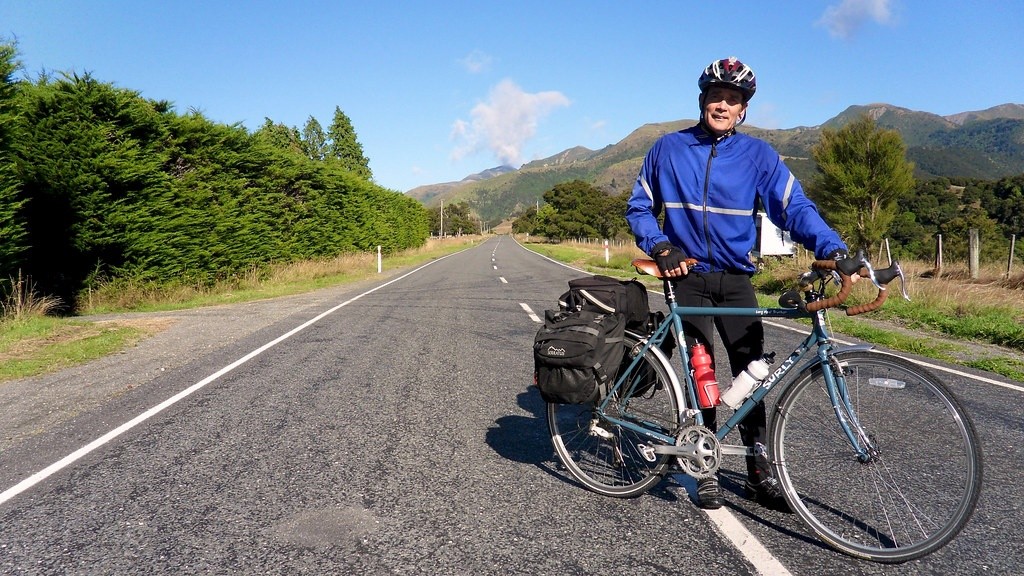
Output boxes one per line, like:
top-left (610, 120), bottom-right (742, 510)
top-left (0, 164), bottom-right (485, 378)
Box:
top-left (721, 351), bottom-right (776, 407)
top-left (691, 337), bottom-right (720, 407)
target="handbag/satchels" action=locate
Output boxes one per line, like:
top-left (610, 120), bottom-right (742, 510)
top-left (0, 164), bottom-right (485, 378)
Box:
top-left (533, 305), bottom-right (626, 404)
top-left (609, 311), bottom-right (676, 398)
top-left (562, 272), bottom-right (651, 331)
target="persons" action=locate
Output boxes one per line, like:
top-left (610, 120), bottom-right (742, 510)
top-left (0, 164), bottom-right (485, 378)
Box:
top-left (625, 57), bottom-right (861, 514)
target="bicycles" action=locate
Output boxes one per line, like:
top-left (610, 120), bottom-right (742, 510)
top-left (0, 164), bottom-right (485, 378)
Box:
top-left (529, 239), bottom-right (984, 566)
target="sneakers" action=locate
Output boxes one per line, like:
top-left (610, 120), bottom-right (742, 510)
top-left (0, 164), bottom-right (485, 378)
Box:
top-left (744, 477), bottom-right (808, 514)
top-left (697, 470), bottom-right (725, 509)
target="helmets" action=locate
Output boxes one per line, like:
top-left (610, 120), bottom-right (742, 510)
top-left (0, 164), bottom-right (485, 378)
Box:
top-left (698, 57), bottom-right (756, 103)
